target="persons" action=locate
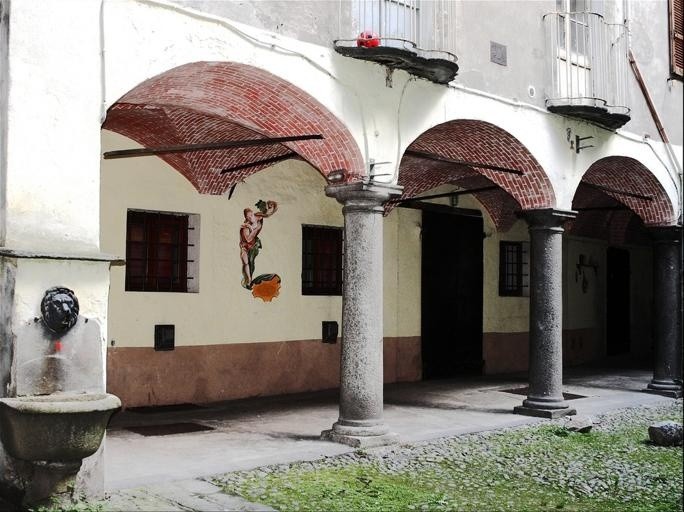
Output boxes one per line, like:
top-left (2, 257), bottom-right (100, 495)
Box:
top-left (240, 200), bottom-right (278, 290)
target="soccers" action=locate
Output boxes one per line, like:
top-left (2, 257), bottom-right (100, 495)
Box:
top-left (357, 32), bottom-right (378, 46)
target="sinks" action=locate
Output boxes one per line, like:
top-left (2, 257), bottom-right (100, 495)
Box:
top-left (0, 392), bottom-right (123, 462)
